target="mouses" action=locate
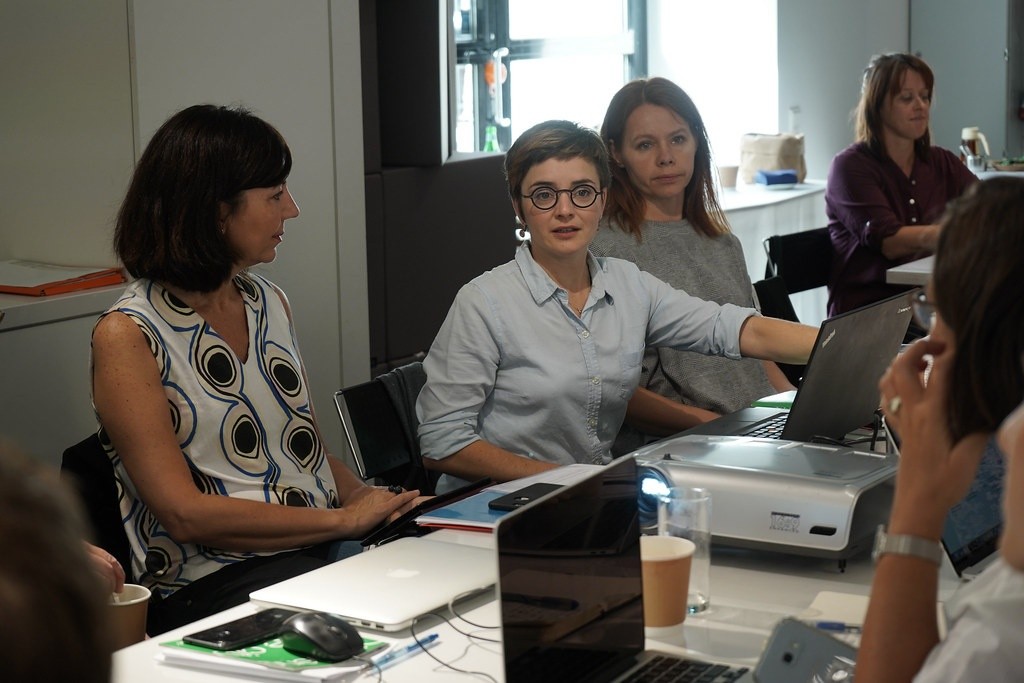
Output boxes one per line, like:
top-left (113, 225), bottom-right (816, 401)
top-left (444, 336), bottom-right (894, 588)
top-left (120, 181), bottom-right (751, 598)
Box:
top-left (281, 611), bottom-right (363, 662)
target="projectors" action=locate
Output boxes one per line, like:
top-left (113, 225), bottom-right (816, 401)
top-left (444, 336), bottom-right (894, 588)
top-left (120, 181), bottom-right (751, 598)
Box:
top-left (633, 436), bottom-right (899, 576)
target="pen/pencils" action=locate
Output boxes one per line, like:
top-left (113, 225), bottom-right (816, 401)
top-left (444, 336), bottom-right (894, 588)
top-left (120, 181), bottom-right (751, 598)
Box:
top-left (808, 621), bottom-right (862, 633)
top-left (364, 634), bottom-right (440, 671)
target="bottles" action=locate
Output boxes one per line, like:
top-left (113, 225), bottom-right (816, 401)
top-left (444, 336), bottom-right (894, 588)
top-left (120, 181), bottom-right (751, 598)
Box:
top-left (483, 126), bottom-right (501, 153)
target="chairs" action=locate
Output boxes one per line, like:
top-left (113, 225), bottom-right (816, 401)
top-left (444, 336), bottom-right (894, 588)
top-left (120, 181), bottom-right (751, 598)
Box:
top-left (326, 362), bottom-right (439, 499)
top-left (57, 433), bottom-right (160, 639)
top-left (762, 226), bottom-right (830, 328)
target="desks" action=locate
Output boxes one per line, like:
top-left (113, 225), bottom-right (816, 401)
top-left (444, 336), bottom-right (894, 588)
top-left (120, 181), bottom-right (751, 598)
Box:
top-left (706, 179), bottom-right (826, 216)
top-left (884, 254), bottom-right (935, 286)
top-left (102, 344), bottom-right (956, 683)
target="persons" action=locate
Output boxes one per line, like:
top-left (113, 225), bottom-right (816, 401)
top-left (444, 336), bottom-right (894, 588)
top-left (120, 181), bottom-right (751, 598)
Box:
top-left (825, 54), bottom-right (981, 320)
top-left (416, 118), bottom-right (821, 500)
top-left (88, 104), bottom-right (436, 637)
top-left (588, 77), bottom-right (796, 453)
top-left (0, 446), bottom-right (125, 683)
top-left (848, 174), bottom-right (1024, 682)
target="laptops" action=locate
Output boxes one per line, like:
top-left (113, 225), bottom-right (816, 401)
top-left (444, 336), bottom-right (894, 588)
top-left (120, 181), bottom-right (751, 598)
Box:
top-left (250, 537), bottom-right (500, 632)
top-left (654, 288), bottom-right (922, 445)
top-left (490, 453), bottom-right (762, 683)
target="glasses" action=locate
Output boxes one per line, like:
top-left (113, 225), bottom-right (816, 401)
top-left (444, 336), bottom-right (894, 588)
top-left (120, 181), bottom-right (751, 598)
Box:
top-left (908, 288), bottom-right (943, 330)
top-left (519, 184), bottom-right (603, 210)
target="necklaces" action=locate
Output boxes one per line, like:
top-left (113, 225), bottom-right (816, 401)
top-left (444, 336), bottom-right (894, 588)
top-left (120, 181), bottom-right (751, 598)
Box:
top-left (569, 302), bottom-right (582, 313)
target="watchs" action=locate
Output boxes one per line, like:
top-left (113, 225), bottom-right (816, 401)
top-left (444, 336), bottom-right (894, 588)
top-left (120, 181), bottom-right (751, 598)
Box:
top-left (871, 524), bottom-right (941, 562)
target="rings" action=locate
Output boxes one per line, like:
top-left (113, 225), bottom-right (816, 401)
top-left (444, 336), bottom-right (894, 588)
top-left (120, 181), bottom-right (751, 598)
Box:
top-left (388, 486), bottom-right (402, 494)
top-left (890, 397), bottom-right (901, 414)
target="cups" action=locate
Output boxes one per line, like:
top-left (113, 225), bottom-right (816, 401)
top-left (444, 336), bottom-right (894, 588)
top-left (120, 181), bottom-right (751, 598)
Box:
top-left (967, 156), bottom-right (985, 172)
top-left (658, 488), bottom-right (710, 614)
top-left (717, 164), bottom-right (739, 192)
top-left (108, 584), bottom-right (151, 653)
top-left (640, 536), bottom-right (696, 627)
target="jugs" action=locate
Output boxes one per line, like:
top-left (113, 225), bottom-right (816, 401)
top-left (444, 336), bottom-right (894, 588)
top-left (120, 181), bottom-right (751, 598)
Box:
top-left (960, 127), bottom-right (990, 163)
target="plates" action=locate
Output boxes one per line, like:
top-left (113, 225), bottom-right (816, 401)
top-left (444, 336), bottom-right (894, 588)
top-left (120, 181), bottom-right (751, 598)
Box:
top-left (992, 162), bottom-right (1024, 171)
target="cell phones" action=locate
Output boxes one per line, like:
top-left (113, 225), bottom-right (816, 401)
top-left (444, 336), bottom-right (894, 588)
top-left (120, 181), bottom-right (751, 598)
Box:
top-left (487, 484), bottom-right (566, 511)
top-left (183, 608), bottom-right (300, 651)
top-left (751, 617), bottom-right (858, 683)
